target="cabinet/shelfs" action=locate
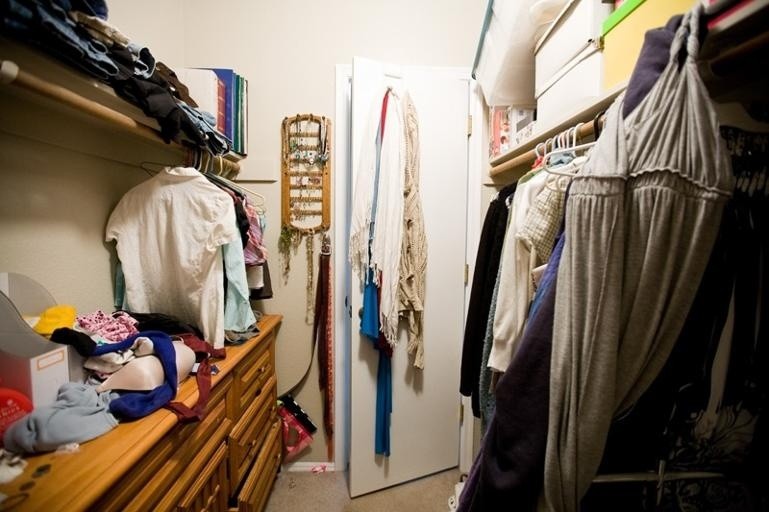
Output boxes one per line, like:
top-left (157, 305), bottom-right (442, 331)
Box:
top-left (1, 370), bottom-right (227, 512)
top-left (227, 314), bottom-right (285, 510)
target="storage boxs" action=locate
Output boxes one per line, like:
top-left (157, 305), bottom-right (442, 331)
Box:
top-left (601, 0), bottom-right (697, 93)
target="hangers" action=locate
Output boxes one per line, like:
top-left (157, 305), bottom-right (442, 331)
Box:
top-left (140, 141), bottom-right (266, 220)
top-left (490, 111), bottom-right (607, 210)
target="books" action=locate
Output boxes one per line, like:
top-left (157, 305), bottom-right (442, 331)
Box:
top-left (183, 68), bottom-right (249, 154)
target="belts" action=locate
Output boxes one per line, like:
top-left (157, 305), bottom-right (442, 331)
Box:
top-left (319, 244), bottom-right (332, 440)
top-left (277, 255), bottom-right (319, 401)
